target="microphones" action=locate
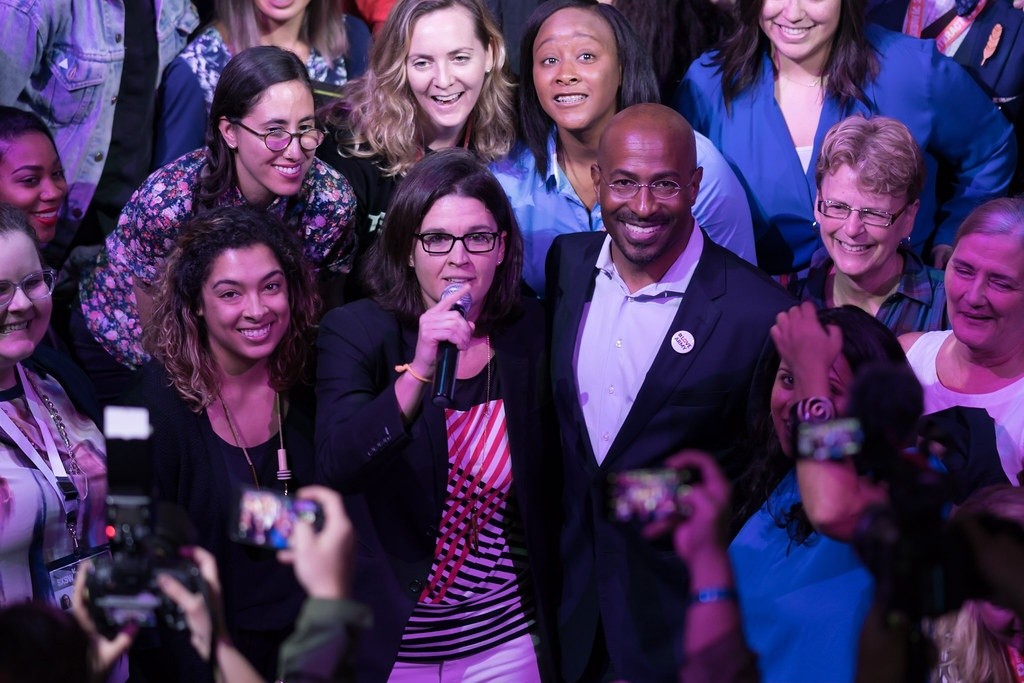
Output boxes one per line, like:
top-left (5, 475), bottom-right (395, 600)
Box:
top-left (430, 284), bottom-right (472, 409)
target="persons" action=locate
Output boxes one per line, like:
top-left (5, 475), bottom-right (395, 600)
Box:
top-left (0, 0), bottom-right (1024, 683)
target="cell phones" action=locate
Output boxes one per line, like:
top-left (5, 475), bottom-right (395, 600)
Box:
top-left (598, 463), bottom-right (700, 525)
top-left (228, 485), bottom-right (324, 552)
top-left (787, 409), bottom-right (870, 463)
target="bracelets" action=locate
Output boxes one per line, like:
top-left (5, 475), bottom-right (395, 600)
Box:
top-left (790, 397), bottom-right (836, 422)
top-left (684, 588), bottom-right (739, 603)
top-left (394, 365), bottom-right (432, 383)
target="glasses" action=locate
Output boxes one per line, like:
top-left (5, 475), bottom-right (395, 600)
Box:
top-left (235, 120), bottom-right (329, 152)
top-left (818, 185), bottom-right (913, 227)
top-left (598, 164), bottom-right (699, 199)
top-left (0, 264), bottom-right (58, 306)
top-left (414, 229), bottom-right (501, 254)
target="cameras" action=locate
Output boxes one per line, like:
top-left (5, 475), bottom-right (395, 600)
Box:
top-left (85, 534), bottom-right (198, 639)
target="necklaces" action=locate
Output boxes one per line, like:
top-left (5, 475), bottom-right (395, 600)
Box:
top-left (0, 365), bottom-right (88, 502)
top-left (216, 362), bottom-right (291, 497)
top-left (444, 332), bottom-right (490, 556)
top-left (776, 66), bottom-right (826, 87)
